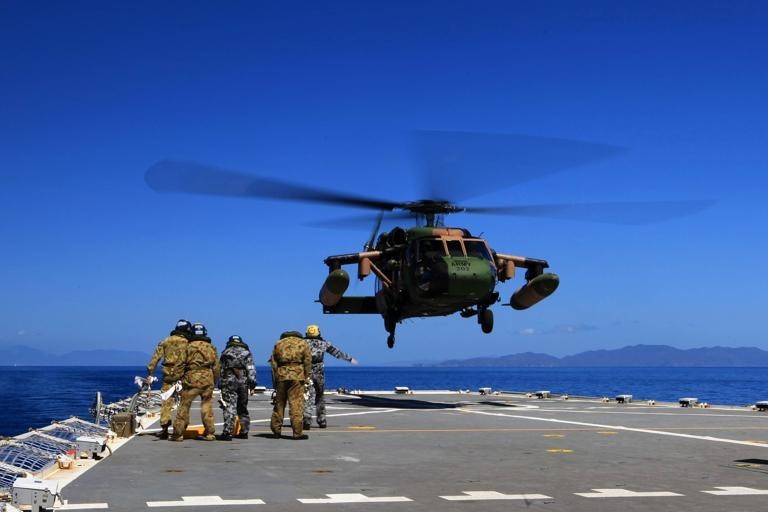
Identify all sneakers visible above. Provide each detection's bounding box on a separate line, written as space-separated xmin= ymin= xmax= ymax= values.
xmin=159 ymin=424 xmax=327 ymax=441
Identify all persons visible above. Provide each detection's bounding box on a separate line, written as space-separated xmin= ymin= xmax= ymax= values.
xmin=218 ymin=335 xmax=257 ymax=441
xmin=143 ymin=318 xmax=192 ymax=440
xmin=303 ymin=325 xmax=357 ymax=429
xmin=169 ymin=323 xmax=220 ymax=442
xmin=271 ymin=331 xmax=312 ymax=439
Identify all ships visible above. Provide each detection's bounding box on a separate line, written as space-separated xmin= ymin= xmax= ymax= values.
xmin=2 ymin=375 xmax=767 ymax=510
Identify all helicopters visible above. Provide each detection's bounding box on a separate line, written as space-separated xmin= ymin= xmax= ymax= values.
xmin=143 ymin=131 xmax=720 ymax=350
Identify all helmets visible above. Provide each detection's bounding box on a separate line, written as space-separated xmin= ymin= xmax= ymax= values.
xmin=176 ymin=319 xmax=320 ymax=342
